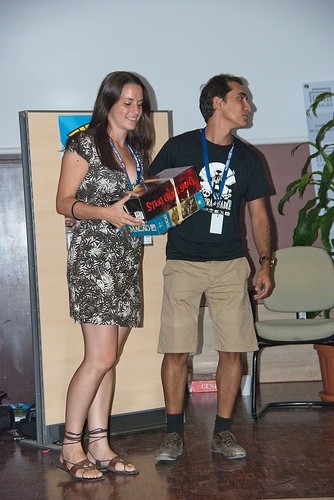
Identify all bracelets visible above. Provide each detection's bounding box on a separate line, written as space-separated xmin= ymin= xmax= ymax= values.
xmin=71 ymin=200 xmax=84 ymax=221
xmin=259 ymin=256 xmax=275 ymax=267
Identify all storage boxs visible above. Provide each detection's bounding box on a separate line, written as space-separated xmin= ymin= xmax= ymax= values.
xmin=188 ymin=373 xmax=218 ymax=393
xmin=109 ymin=165 xmax=201 ymax=229
xmin=126 ymin=191 xmax=205 ymax=236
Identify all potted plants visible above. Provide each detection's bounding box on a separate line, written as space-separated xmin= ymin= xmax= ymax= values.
xmin=278 ymin=92 xmax=334 ymax=408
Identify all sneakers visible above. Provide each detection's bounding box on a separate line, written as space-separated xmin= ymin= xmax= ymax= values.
xmin=153 ymin=433 xmax=183 ymax=460
xmin=210 ymin=431 xmax=246 ymax=460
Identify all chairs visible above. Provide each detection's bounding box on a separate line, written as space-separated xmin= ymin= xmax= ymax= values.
xmin=251 ymin=246 xmax=334 ymax=420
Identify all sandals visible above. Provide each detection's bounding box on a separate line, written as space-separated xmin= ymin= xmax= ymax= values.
xmin=86 ymin=429 xmax=139 ymax=475
xmin=56 ymin=430 xmax=106 ymax=481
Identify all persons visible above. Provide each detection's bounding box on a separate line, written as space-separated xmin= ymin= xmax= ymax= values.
xmin=56 ymin=71 xmax=156 ymax=482
xmin=145 ymin=74 xmax=277 ymax=460
